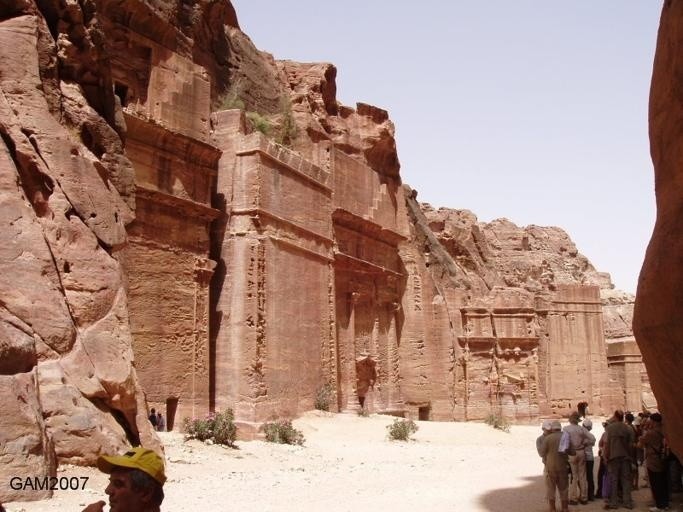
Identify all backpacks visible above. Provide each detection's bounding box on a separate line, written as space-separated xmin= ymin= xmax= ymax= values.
xmin=650 ymin=430 xmax=672 ymax=460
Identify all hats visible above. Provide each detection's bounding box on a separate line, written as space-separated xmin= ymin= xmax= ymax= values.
xmin=568 ymin=412 xmax=583 ymax=422
xmin=542 ymin=421 xmax=552 ymax=430
xmin=552 ymin=420 xmax=561 ymax=429
xmin=651 ymin=413 xmax=662 ymax=422
xmin=583 ymin=419 xmax=592 ymax=430
xmin=97 ymin=448 xmax=166 ymax=487
xmin=602 ymin=419 xmax=614 ymax=427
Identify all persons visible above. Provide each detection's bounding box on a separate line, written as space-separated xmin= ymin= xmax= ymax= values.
xmin=149 ymin=408 xmax=157 ymax=426
xmin=156 ymin=413 xmax=166 ymax=432
xmin=536 ymin=401 xmax=682 ymax=512
xmin=82 ymin=447 xmax=167 ymax=512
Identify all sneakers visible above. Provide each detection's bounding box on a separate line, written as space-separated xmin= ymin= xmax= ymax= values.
xmin=604 ymin=502 xmax=633 ymax=510
xmin=632 ymin=484 xmax=651 ymax=490
xmin=650 ymin=503 xmax=672 ymax=512
xmin=569 ymin=495 xmax=602 ymax=504
